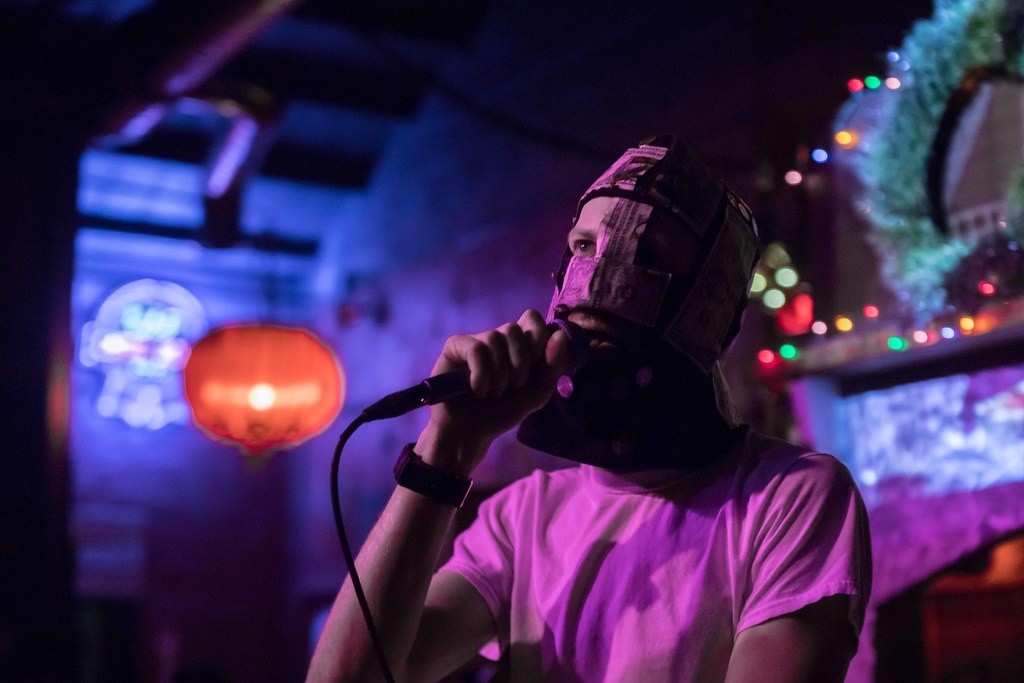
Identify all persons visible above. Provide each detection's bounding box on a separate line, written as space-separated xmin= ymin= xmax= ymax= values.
xmin=305 ymin=133 xmax=873 ymax=683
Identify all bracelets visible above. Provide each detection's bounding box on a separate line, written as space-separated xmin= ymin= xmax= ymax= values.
xmin=393 ymin=443 xmax=473 ymax=507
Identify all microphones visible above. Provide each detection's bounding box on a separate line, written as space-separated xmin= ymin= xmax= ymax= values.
xmin=360 ymin=320 xmax=585 ymax=423
